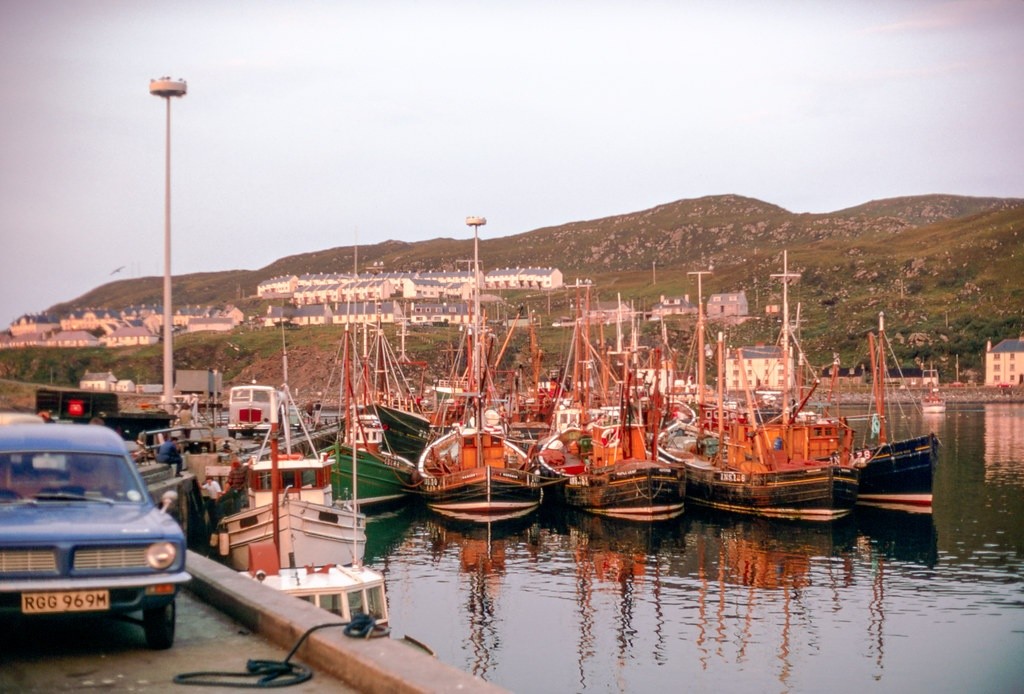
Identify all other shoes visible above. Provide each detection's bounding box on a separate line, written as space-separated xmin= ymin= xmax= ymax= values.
xmin=175 ymin=474 xmax=183 ymax=477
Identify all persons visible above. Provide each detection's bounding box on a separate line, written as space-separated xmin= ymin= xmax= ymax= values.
xmin=180 ymin=403 xmax=195 ymax=438
xmin=306 ymin=400 xmax=321 ymax=422
xmin=156 ymin=436 xmax=184 ymax=477
xmin=198 ymin=459 xmax=252 ymax=499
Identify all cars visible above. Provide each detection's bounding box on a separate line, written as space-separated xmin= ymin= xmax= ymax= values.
xmin=1 ymin=420 xmax=191 ymax=652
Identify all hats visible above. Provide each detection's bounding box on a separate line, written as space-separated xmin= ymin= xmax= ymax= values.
xmin=232 ymin=462 xmax=241 ymax=468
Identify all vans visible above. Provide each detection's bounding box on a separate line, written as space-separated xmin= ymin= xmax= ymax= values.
xmin=226 ymin=384 xmax=288 ymax=439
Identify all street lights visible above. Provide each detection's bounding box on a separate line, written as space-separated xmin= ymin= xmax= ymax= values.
xmin=464 ymin=215 xmax=489 ymax=380
xmin=149 ymin=74 xmax=189 ymax=422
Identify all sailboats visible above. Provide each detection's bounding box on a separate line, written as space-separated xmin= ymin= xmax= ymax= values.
xmin=205 ymin=250 xmax=940 ymax=634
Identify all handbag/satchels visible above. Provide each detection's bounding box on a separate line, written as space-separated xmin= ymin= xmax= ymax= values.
xmin=222 ymin=488 xmax=241 ymax=516
